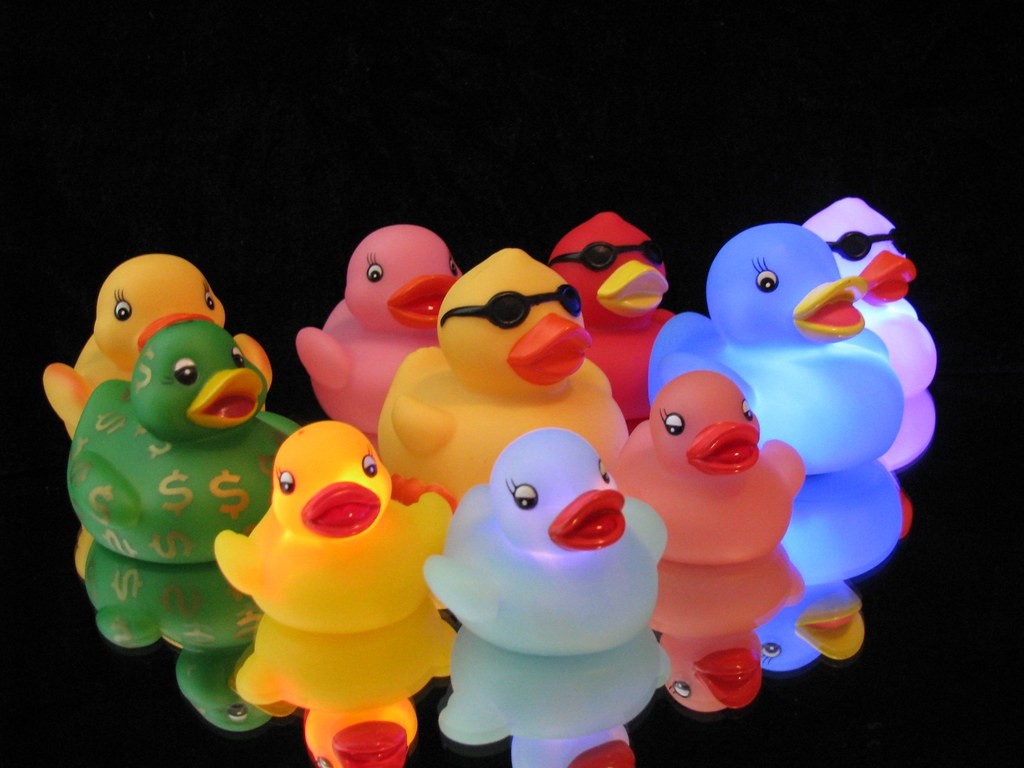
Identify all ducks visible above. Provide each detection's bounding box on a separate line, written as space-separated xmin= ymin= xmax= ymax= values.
xmin=41 ymin=193 xmax=939 ymax=660
xmin=176 ymin=581 xmax=867 ymax=768
